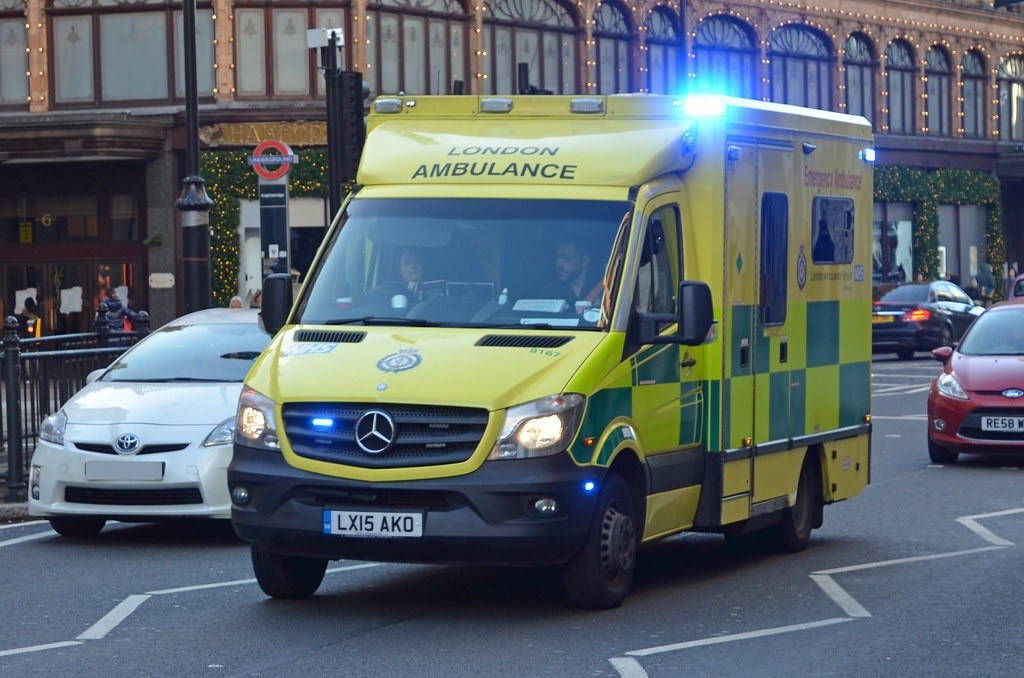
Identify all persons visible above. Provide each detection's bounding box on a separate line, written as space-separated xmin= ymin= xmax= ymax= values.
xmin=230 ymin=296 xmax=242 ymax=308
xmin=18 ymin=297 xmax=39 ymax=383
xmin=398 ymin=249 xmax=443 ymax=301
xmin=1005 ymin=268 xmax=1020 ymax=300
xmin=249 ymin=289 xmax=262 ymax=308
xmin=962 ymin=265 xmax=999 ymax=308
xmin=96 ymin=287 xmax=137 ymax=347
xmin=555 ymin=239 xmax=603 ymax=328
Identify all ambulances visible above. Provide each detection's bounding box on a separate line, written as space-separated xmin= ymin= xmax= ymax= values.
xmin=227 ymin=93 xmax=875 ymax=613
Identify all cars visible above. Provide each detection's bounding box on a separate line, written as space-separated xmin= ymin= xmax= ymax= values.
xmin=925 ymin=298 xmax=1023 ymax=465
xmin=27 ymin=309 xmax=273 ymax=539
xmin=872 ymin=280 xmax=985 ymax=362
